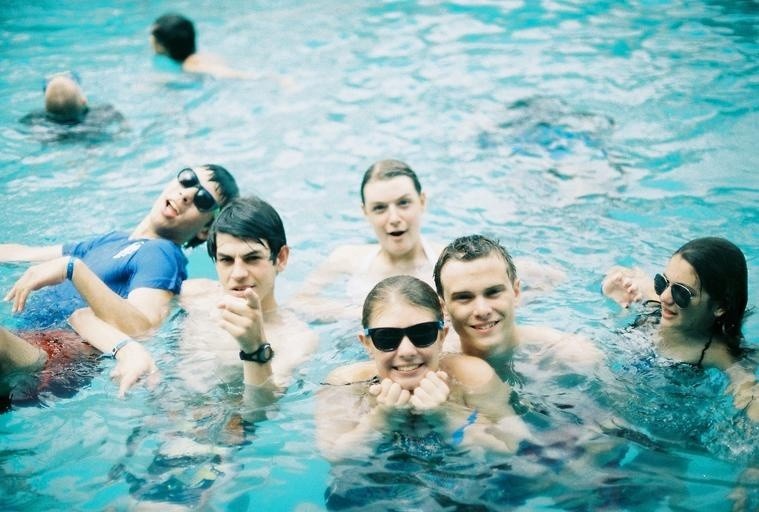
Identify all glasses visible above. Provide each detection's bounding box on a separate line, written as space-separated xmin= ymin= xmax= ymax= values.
xmin=654 ymin=274 xmax=693 ymax=309
xmin=179 ymin=169 xmax=219 ymax=212
xmin=365 ymin=321 xmax=444 ymax=353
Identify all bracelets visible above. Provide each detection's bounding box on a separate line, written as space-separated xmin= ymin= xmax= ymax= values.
xmin=65 ymin=255 xmax=81 ymax=282
xmin=445 ymin=407 xmax=479 ymax=449
xmin=94 ymin=337 xmax=136 ymax=360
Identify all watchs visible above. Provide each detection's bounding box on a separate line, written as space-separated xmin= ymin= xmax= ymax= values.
xmin=239 ymin=341 xmax=275 ymax=365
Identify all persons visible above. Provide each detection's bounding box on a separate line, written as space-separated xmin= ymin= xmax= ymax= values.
xmin=316 ymin=272 xmax=531 ymax=510
xmin=69 ymin=197 xmax=318 ymax=512
xmin=591 ymin=236 xmax=759 ymax=511
xmin=430 ymin=234 xmax=632 ymax=511
xmin=290 ymin=161 xmax=570 ymax=331
xmin=0 ymin=163 xmax=237 ymax=416
xmin=152 ymin=12 xmax=257 ymax=80
xmin=22 ymin=70 xmax=136 ymax=147
